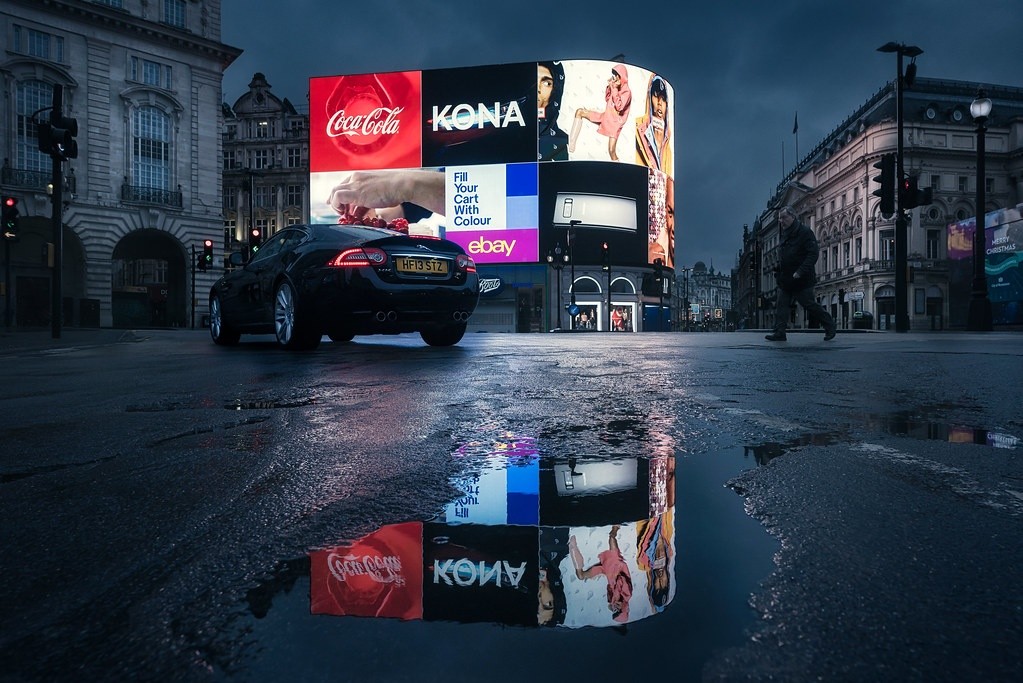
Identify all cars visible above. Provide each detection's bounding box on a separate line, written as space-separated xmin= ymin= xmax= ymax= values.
xmin=207 ymin=223 xmax=481 ymax=351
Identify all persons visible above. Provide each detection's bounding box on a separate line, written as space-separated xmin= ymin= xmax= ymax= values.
xmin=326 ymin=166 xmax=446 ymax=229
xmin=765 ymin=207 xmax=838 ymax=341
xmin=575 ymin=308 xmax=596 ymax=330
xmin=538 ymin=507 xmax=674 ymax=627
xmin=537 ymin=60 xmax=672 ymax=175
xmin=648 ymin=175 xmax=674 ymax=268
xmin=611 ymin=307 xmax=628 ymax=331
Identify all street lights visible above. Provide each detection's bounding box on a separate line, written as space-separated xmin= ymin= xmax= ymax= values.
xmin=545 ymin=242 xmax=570 ymax=333
xmin=682 ymin=267 xmax=695 ymax=331
xmin=876 ymin=41 xmax=925 ymax=336
xmin=601 ymin=242 xmax=611 ymax=332
xmin=960 ymin=84 xmax=996 ymax=333
xmin=568 ymin=219 xmax=582 ymax=330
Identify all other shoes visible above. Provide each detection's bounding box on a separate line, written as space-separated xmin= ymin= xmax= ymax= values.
xmin=824 ymin=323 xmax=838 ymax=340
xmin=765 ymin=332 xmax=788 ymax=341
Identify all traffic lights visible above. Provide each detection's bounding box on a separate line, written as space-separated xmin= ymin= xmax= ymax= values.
xmin=197 ymin=254 xmax=206 ymax=271
xmin=687 ymin=300 xmax=692 ymax=310
xmin=2 ymin=196 xmax=20 ymax=233
xmin=872 ymin=152 xmax=895 ymax=215
xmin=39 ymin=122 xmax=70 ymax=156
xmin=204 ymin=240 xmax=214 ymax=271
xmin=899 ymin=177 xmax=921 ymax=208
xmin=250 ymin=229 xmax=260 ymax=257
xmin=42 ymin=243 xmax=54 ymax=269
xmin=55 ymin=116 xmax=79 ymax=161
xmin=242 ymin=244 xmax=249 ymax=263
xmin=654 ymin=258 xmax=662 ymax=282
